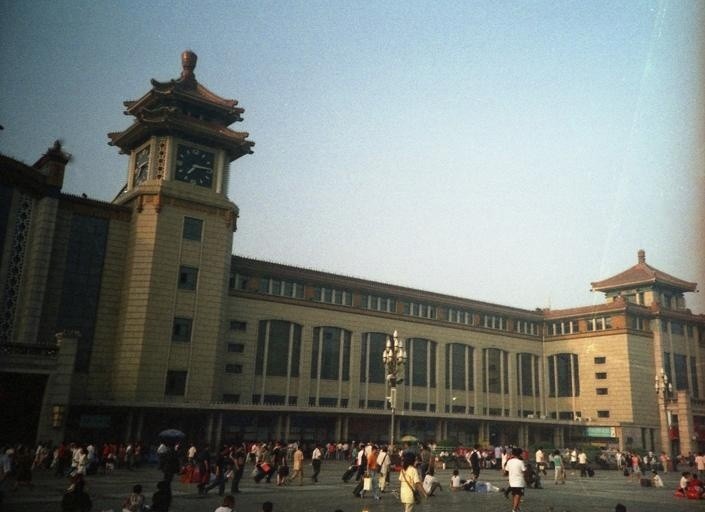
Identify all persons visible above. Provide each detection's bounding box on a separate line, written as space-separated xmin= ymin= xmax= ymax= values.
xmin=311 ymin=440 xmax=592 ymax=511
xmin=597 ymin=446 xmax=704 ymax=511
xmin=2 ymin=436 xmax=304 ymax=511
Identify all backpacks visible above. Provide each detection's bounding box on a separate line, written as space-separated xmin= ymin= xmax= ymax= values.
xmin=470 ymin=450 xmax=480 ymax=465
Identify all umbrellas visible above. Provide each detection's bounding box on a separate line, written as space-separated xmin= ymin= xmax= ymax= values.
xmin=158 ymin=429 xmax=185 ymax=441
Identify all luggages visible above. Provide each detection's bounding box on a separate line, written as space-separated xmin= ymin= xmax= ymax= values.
xmin=342 ymin=469 xmax=353 ymax=482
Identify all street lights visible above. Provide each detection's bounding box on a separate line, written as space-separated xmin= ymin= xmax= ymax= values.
xmin=652 ymin=366 xmax=678 ymax=470
xmin=380 ymin=329 xmax=408 ymax=465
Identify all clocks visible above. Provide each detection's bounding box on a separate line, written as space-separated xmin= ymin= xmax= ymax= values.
xmin=133 ymin=145 xmax=149 ymax=187
xmin=175 ymin=146 xmax=215 ymax=187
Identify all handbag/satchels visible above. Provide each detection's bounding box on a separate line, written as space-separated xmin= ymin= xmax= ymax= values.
xmin=278 ymin=465 xmax=289 ymax=476
xmin=413 ymin=489 xmax=422 ymax=505
xmin=362 ymin=477 xmax=373 ymax=491
xmin=376 ymin=464 xmax=381 ymax=474
